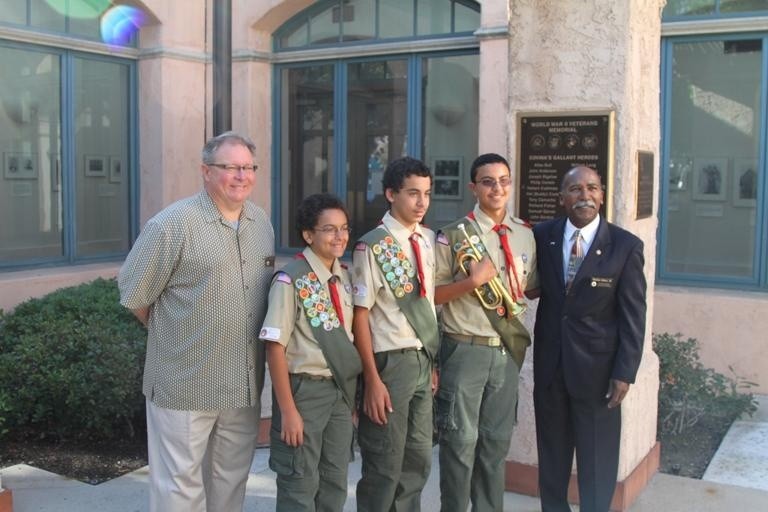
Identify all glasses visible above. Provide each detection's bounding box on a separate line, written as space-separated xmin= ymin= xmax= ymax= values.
xmin=476 ymin=179 xmax=512 ymax=187
xmin=206 ymin=163 xmax=258 ymax=172
xmin=315 ymin=227 xmax=352 ymax=235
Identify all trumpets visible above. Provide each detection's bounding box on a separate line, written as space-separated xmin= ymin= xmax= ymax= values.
xmin=457 ymin=224 xmax=527 ymax=321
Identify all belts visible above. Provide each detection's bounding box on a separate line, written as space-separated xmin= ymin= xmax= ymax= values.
xmin=444 ymin=332 xmax=504 ymax=347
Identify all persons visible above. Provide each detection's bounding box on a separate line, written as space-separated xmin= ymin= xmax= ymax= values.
xmin=116 ymin=131 xmax=276 ymax=512
xmin=351 ymin=155 xmax=440 ymax=511
xmin=256 ymin=192 xmax=360 ymax=511
xmin=433 ymin=153 xmax=542 ymax=512
xmin=526 ymin=163 xmax=647 ymax=511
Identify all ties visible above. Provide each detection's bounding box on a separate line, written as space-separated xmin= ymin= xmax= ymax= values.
xmin=565 ymin=230 xmax=585 ymax=296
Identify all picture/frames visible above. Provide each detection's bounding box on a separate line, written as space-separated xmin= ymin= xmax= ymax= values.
xmin=694 ymin=156 xmax=759 ymax=208
xmin=3 ymin=151 xmax=61 ymax=193
xmin=429 ymin=156 xmax=463 ymax=200
xmin=84 ymin=154 xmax=123 ymax=183
